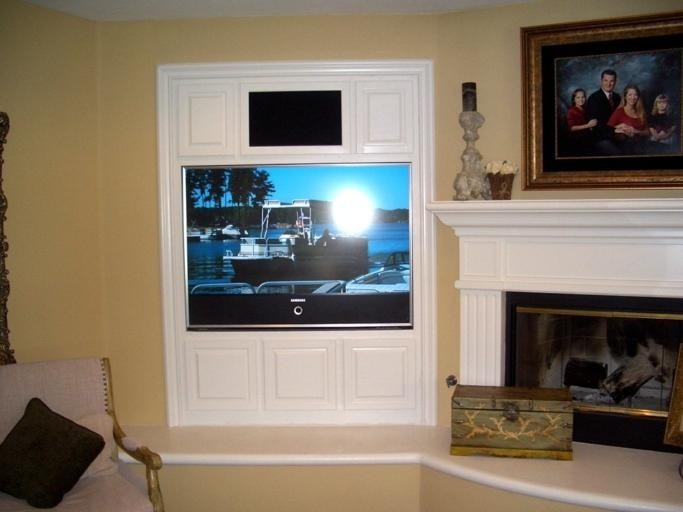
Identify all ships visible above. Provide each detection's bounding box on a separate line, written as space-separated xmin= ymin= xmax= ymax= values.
xmin=221 ymin=201 xmax=368 ymax=286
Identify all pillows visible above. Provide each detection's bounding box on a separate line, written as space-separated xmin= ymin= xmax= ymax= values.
xmin=0 ymin=397 xmax=114 ymax=509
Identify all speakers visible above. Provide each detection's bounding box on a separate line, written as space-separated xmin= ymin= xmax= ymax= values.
xmin=247 ymin=90 xmax=344 ymax=148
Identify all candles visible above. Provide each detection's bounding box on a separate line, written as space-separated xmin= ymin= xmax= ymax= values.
xmin=462 ymin=82 xmax=477 ymax=111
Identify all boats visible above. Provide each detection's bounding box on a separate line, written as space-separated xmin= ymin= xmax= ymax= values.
xmin=189 ymin=221 xmax=248 ymax=239
xmin=345 ymin=251 xmax=409 ymax=294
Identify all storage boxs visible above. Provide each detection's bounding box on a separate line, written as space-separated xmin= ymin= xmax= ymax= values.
xmin=450 ymin=381 xmax=573 ymax=460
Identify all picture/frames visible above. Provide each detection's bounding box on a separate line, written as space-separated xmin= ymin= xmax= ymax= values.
xmin=520 ymin=9 xmax=683 ymax=190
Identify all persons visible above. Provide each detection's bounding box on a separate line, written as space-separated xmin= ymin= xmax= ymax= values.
xmin=565 ymin=69 xmax=676 ymax=157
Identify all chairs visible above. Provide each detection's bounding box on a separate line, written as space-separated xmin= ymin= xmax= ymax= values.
xmin=0 ymin=358 xmax=164 ymax=512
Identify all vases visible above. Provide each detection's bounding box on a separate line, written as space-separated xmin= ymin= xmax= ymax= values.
xmin=487 ymin=174 xmax=514 ymax=200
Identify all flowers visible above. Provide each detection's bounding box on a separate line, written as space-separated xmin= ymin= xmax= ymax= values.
xmin=482 ymin=159 xmax=518 ymax=181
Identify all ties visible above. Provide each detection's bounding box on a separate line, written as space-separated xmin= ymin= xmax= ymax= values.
xmin=608 ymin=93 xmax=613 ymax=108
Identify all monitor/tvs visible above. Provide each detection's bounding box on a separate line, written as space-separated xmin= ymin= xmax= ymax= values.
xmin=182 ymin=161 xmax=414 ymax=332
